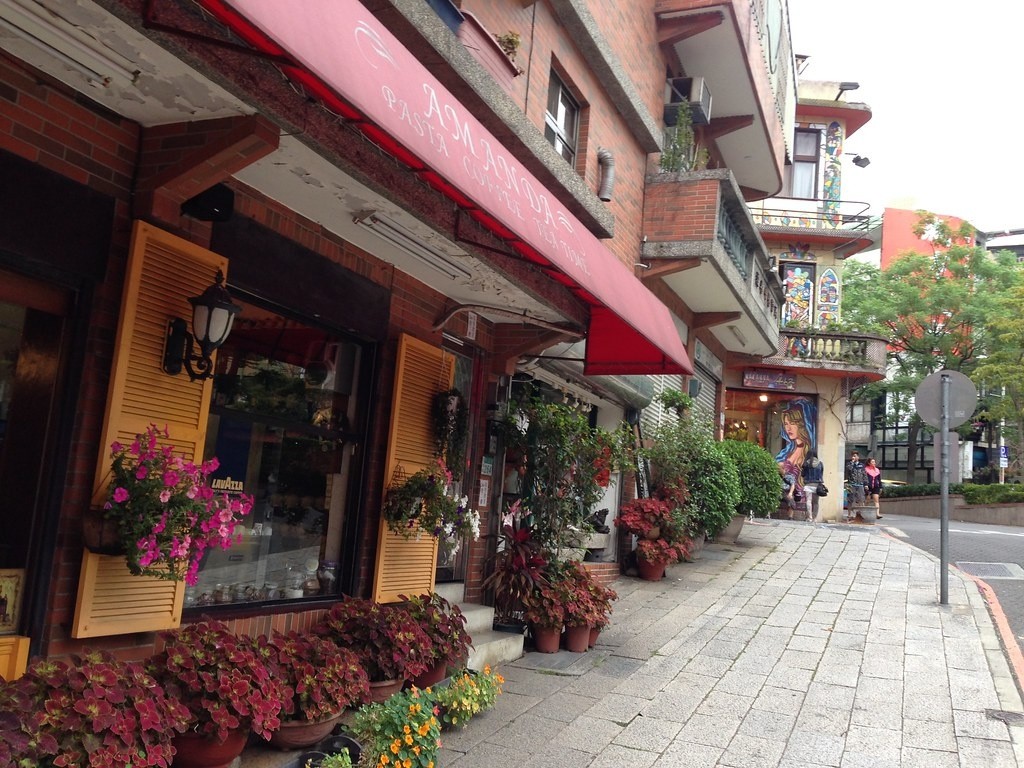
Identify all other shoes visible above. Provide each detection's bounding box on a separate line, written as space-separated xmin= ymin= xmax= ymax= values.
xmin=846 ymin=516 xmax=853 ymax=519
xmin=876 ymin=515 xmax=883 ymax=519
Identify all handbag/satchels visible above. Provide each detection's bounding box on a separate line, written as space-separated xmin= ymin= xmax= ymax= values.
xmin=793 ymin=488 xmax=801 ymax=502
xmin=816 ymin=483 xmax=828 ymax=496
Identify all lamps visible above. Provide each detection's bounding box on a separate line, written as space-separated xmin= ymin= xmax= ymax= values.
xmin=162 ymin=267 xmax=241 ymax=384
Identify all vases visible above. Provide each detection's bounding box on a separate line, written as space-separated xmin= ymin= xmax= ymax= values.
xmin=405 ymin=495 xmax=424 ymax=519
xmin=80 ymin=504 xmax=131 ymax=557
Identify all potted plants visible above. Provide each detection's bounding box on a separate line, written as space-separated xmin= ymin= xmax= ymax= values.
xmin=713 ymin=440 xmax=784 ymax=543
xmin=525 ymin=559 xmax=619 ymax=653
xmin=679 ymin=436 xmax=741 ymax=559
xmin=148 ymin=619 xmax=278 ymax=768
xmin=456 ymin=10 xmax=525 ymax=96
xmin=315 ymin=592 xmax=433 ymax=706
xmin=396 ymin=590 xmax=475 ymax=685
xmin=479 ymin=497 xmax=546 ymax=633
xmin=243 ymin=627 xmax=371 ymax=749
xmin=635 ymin=540 xmax=677 ymax=581
xmin=431 ymin=387 xmax=469 ymax=457
xmin=613 ymin=497 xmax=670 ymax=540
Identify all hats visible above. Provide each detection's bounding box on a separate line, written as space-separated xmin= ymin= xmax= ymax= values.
xmin=851 ymin=450 xmax=859 ymax=455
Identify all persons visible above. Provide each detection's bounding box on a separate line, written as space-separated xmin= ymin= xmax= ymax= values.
xmin=846 ymin=450 xmax=870 ymax=520
xmin=779 ymin=462 xmax=797 ymax=520
xmin=865 ymin=458 xmax=883 ymax=519
xmin=802 ymin=448 xmax=824 ymax=522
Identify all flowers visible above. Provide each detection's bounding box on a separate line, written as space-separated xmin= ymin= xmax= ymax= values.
xmin=110 ymin=421 xmax=253 ymax=585
xmin=305 ymin=662 xmax=504 ymax=768
xmin=384 ymin=459 xmax=481 ymax=559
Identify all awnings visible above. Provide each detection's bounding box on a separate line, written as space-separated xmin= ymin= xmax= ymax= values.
xmin=198 ymin=0 xmax=695 ymax=376
